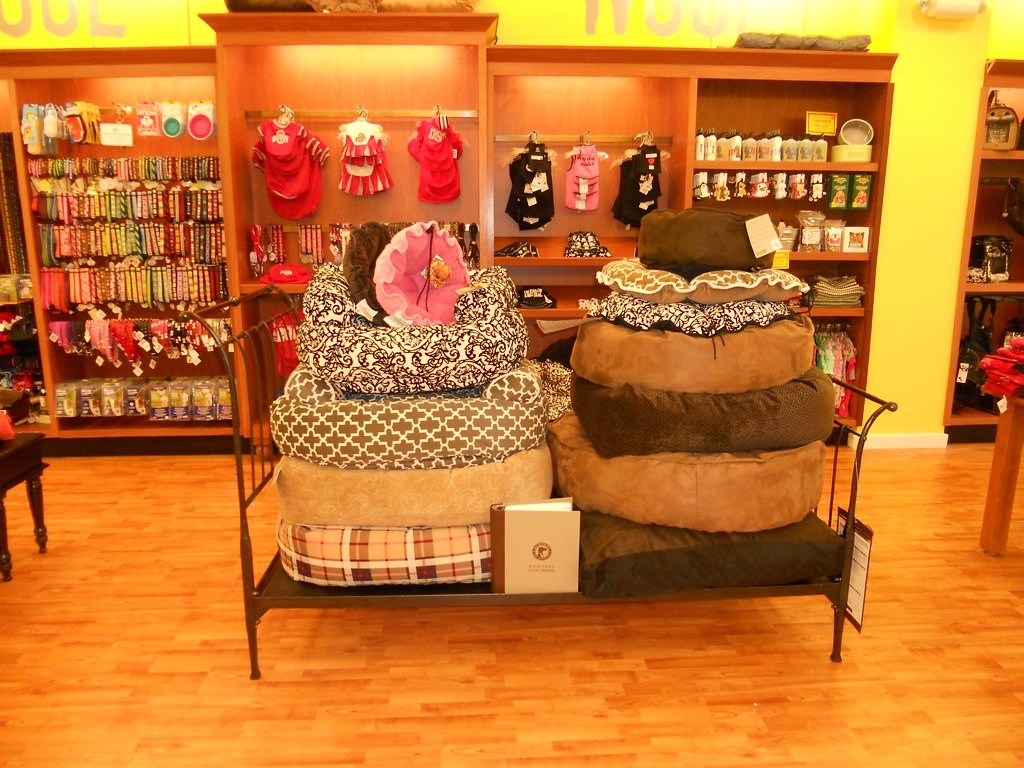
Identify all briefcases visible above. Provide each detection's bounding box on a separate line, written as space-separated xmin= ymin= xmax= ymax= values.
xmin=967 ymin=234 xmax=1012 ymax=283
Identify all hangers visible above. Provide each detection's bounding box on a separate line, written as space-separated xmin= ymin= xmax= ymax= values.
xmin=414 ymin=104 xmax=441 ymax=126
xmin=498 ymin=131 xmax=673 ymax=170
xmin=276 ymin=106 xmax=296 ymax=125
xmin=339 ymin=105 xmax=384 ymax=133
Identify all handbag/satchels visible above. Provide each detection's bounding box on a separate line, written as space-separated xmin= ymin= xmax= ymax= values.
xmin=1002 ymin=178 xmax=1024 ymax=235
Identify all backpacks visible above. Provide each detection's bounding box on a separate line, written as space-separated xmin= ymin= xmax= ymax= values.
xmin=954 ymin=295 xmax=1000 ymax=415
xmin=982 ymin=90 xmax=1020 ymax=151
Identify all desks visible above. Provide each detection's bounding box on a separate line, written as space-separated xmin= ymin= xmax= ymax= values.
xmin=0 ymin=433 xmax=50 ymax=583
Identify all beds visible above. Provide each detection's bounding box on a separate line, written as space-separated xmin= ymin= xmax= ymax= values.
xmin=178 ymin=284 xmax=897 ymax=680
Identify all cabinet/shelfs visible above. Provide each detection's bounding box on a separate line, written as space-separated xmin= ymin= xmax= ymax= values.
xmin=486 ymin=45 xmax=899 ymax=448
xmin=198 ymin=12 xmax=499 ymax=452
xmin=943 ymin=57 xmax=1024 ymax=443
xmin=0 ymin=46 xmax=246 ymax=444
